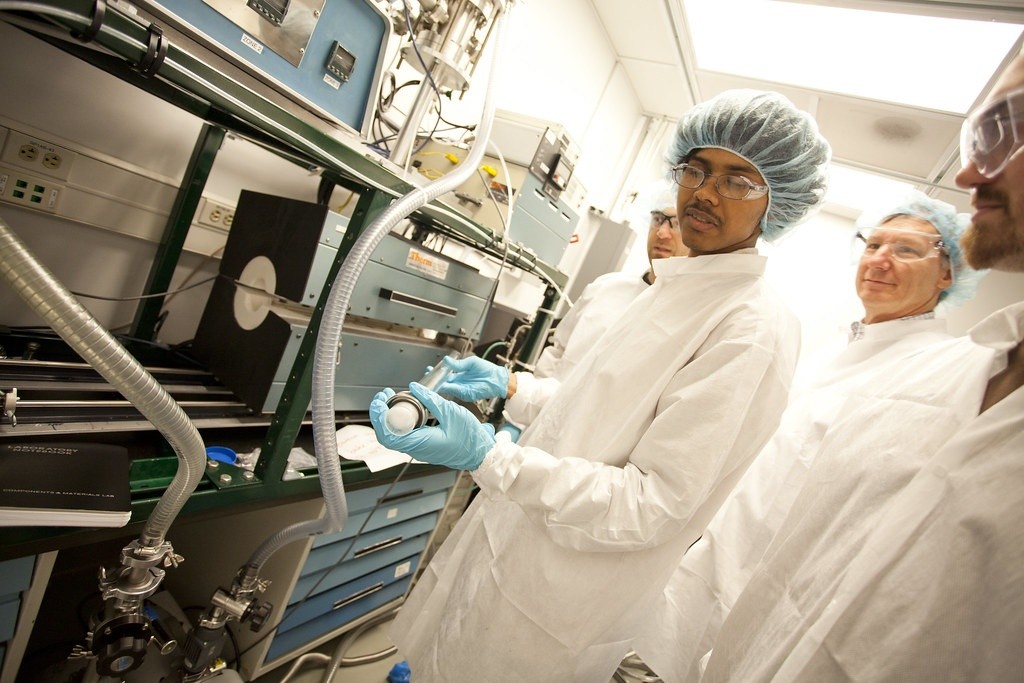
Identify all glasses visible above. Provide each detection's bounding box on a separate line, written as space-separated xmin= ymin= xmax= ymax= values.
xmin=670 ymin=163 xmax=768 ymax=200
xmin=648 ymin=211 xmax=681 ymax=233
xmin=959 ymin=89 xmax=1024 ymax=178
xmin=854 ymin=225 xmax=951 ymax=263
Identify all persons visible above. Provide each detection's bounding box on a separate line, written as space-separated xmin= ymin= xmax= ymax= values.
xmin=698 ymin=46 xmax=1024 ymax=683
xmin=495 ymin=198 xmax=691 ymax=443
xmin=368 ymin=90 xmax=827 ymax=682
xmin=615 ymin=198 xmax=968 ymax=682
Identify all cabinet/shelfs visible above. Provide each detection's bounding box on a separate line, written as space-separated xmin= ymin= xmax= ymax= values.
xmin=0 ymin=1 xmax=573 ymax=683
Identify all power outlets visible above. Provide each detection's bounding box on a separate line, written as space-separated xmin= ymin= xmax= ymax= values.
xmin=197 ymin=199 xmax=235 ymax=231
xmin=2 ymin=130 xmax=78 ymax=181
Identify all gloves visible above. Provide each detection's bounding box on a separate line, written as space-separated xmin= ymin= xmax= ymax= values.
xmin=423 ymin=354 xmax=509 ymax=401
xmin=369 ymin=381 xmax=495 ymax=472
